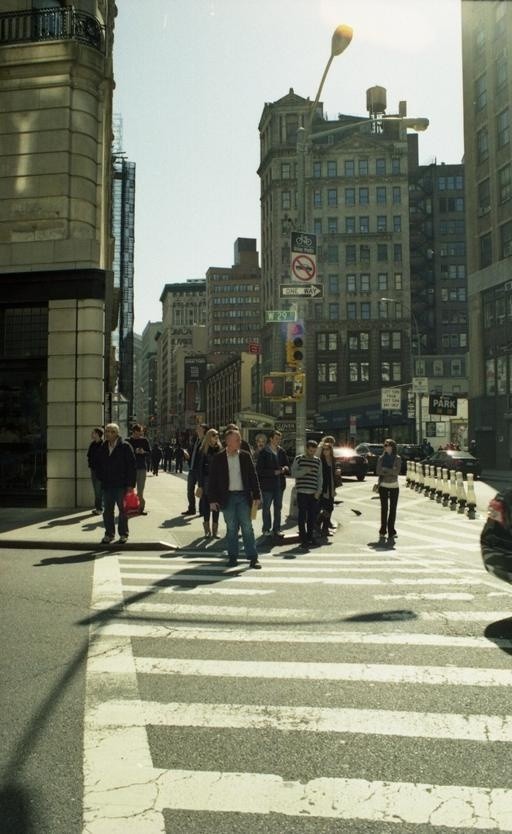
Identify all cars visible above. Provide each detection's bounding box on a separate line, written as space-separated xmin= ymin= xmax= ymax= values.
xmin=418 ymin=449 xmax=482 ymax=479
xmin=480 ymin=488 xmax=512 ymax=585
xmin=332 ymin=444 xmax=369 ymax=482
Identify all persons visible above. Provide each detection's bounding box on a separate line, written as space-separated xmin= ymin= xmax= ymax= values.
xmin=376 ymin=438 xmax=402 ymax=536
xmin=86 ymin=423 xmax=337 ymax=569
xmin=421 ymin=441 xmax=461 ymax=460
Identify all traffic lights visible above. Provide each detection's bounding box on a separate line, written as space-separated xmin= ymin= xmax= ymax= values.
xmin=286 ymin=321 xmax=304 ymax=366
xmin=261 ymin=371 xmax=288 ymax=400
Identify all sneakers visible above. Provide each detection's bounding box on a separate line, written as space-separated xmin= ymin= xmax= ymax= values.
xmin=227 ymin=557 xmax=237 ymax=566
xmin=262 ymin=528 xmax=284 ymax=537
xmin=119 ymin=535 xmax=128 ymax=542
xmin=249 ymin=560 xmax=262 ymax=569
xmin=102 ymin=535 xmax=113 ymax=542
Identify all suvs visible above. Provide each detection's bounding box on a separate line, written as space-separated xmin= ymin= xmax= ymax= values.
xmin=393 ymin=443 xmax=427 ymax=475
xmin=351 ymin=441 xmax=384 ymax=475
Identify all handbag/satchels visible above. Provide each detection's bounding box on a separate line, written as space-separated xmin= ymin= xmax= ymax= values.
xmin=123 ymin=493 xmax=141 ymax=517
xmin=373 ymin=484 xmax=378 ymax=493
xmin=93 ymin=510 xmax=102 ymax=515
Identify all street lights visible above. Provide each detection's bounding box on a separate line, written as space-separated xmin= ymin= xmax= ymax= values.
xmin=379 ymin=295 xmax=425 ymax=444
xmin=287 ymin=16 xmax=431 ymax=456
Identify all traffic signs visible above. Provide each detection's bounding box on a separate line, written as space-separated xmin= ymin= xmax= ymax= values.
xmin=273 ymin=419 xmax=315 ymax=432
xmin=279 ymin=283 xmax=326 ymax=299
xmin=264 ymin=309 xmax=298 ymax=323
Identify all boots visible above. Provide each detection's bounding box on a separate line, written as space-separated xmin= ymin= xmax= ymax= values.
xmin=202 ymin=521 xmax=220 ymax=539
